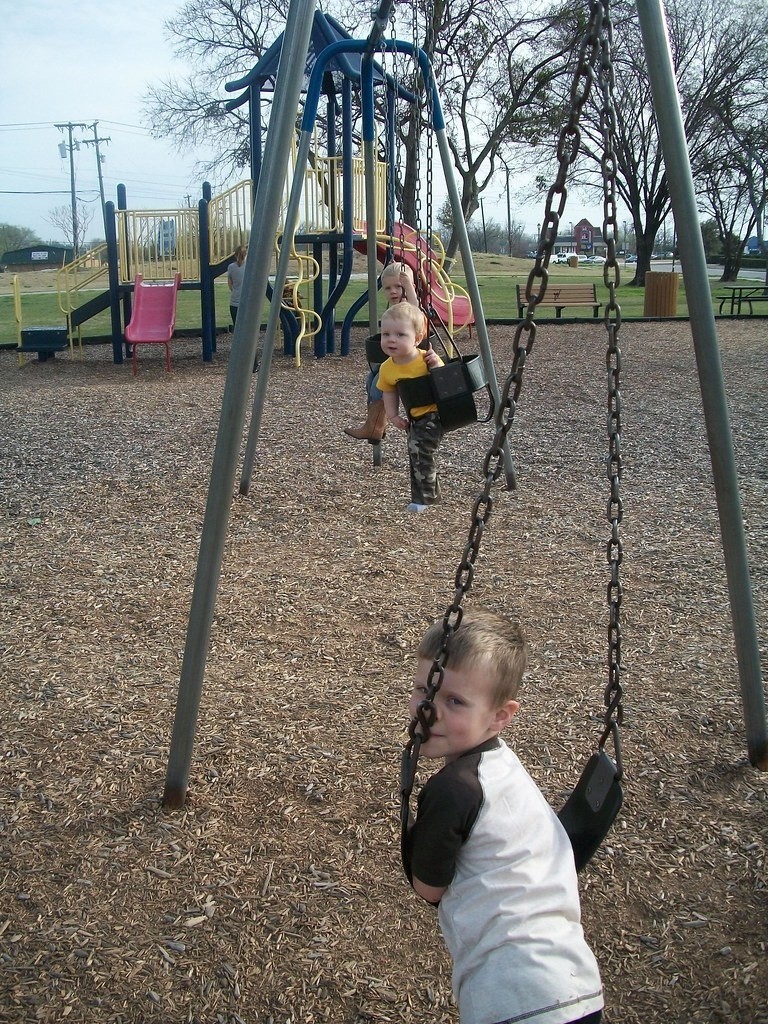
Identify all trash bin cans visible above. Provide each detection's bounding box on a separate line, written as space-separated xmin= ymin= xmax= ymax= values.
xmin=570 ymin=255 xmax=579 ymax=268
xmin=643 ymin=270 xmax=679 ymax=318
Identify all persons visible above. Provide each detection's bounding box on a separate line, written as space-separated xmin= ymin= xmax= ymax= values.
xmin=400 ymin=610 xmax=603 ymax=1024
xmin=282 ymin=279 xmax=302 ymax=332
xmin=227 ymin=245 xmax=260 ymax=373
xmin=344 ymin=261 xmax=445 ymax=513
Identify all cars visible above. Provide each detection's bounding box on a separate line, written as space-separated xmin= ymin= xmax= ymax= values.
xmin=585 ymin=255 xmax=605 ymax=263
xmin=626 ymin=256 xmax=639 ymax=263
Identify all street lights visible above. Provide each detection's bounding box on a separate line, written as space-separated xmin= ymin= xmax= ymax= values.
xmin=569 ymin=221 xmax=573 ymax=252
xmin=623 ymin=220 xmax=627 ymax=259
xmin=537 ymin=223 xmax=541 ymax=250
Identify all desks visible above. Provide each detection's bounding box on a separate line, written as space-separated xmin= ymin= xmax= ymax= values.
xmin=716 ymin=286 xmax=768 ymax=314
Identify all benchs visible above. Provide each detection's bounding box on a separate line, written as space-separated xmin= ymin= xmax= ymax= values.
xmin=516 ymin=283 xmax=603 ymax=318
xmin=716 ymin=296 xmax=768 ymax=301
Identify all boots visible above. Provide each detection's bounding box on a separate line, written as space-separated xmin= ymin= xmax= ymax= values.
xmin=344 ymin=399 xmax=387 ymax=446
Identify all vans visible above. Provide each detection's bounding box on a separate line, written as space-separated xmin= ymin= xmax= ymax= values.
xmin=574 ymin=255 xmax=588 ymax=261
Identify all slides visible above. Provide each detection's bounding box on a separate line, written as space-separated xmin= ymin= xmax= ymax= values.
xmin=353 ymin=224 xmax=476 ymax=328
xmin=124 ymin=274 xmax=178 ymax=344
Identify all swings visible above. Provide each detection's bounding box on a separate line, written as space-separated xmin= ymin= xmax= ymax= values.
xmin=368 ymin=2 xmax=496 ymax=431
xmin=396 ymin=0 xmax=629 ymax=909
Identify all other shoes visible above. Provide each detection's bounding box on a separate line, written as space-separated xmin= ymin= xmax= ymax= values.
xmin=253 ymin=367 xmax=259 ymax=373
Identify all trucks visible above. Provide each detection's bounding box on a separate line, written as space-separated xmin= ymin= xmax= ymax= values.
xmin=541 ymin=253 xmax=577 ymax=263
